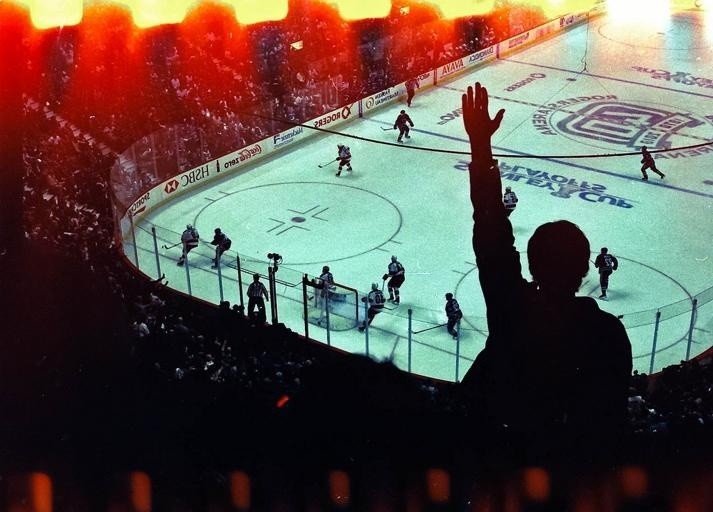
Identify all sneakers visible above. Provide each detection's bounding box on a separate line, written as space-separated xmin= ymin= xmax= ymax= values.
xmin=661 ymin=174 xmax=664 ymax=179
xmin=642 ymin=177 xmax=648 ymax=180
xmin=359 ymin=327 xmax=365 ymax=331
xmin=599 ymin=294 xmax=606 ymax=297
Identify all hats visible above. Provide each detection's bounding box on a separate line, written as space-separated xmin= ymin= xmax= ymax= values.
xmin=253 ymin=273 xmax=261 ymax=278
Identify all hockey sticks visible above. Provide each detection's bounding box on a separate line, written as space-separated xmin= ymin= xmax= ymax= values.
xmin=165 ymin=242 xmax=181 ymax=250
xmin=385 ymin=306 xmax=398 ymax=311
xmin=410 ymin=323 xmax=447 ymax=335
xmin=381 ymin=127 xmax=394 ymax=131
xmin=318 ymin=160 xmax=337 ymax=168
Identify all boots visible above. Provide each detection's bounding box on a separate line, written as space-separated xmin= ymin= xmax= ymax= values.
xmin=347 ymin=167 xmax=352 ymax=170
xmin=336 ymin=173 xmax=340 ymax=176
xmin=398 ymin=137 xmax=403 ymax=142
xmin=405 ymin=133 xmax=410 ymax=138
xmin=178 ymin=261 xmax=184 ymax=265
xmin=389 ymin=297 xmax=399 ymax=303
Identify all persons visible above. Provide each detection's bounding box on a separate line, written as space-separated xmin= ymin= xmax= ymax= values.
xmin=1 ymin=184 xmax=152 ymax=510
xmin=1 ymin=0 xmax=558 ymax=184
xmin=502 ymin=185 xmax=519 ymax=219
xmin=318 ymin=264 xmax=334 ymax=308
xmin=639 ymin=143 xmax=665 ymax=182
xmin=174 ymin=223 xmax=199 ymax=266
xmin=153 ymin=273 xmax=712 ymax=512
xmin=593 ymin=247 xmax=617 ymax=299
xmin=382 ymin=254 xmax=405 ymax=303
xmin=208 ymin=227 xmax=232 ymax=269
xmin=452 ymin=79 xmax=632 ymax=470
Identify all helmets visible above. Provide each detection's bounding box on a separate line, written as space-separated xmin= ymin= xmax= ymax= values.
xmin=215 ymin=228 xmax=220 ymax=233
xmin=187 ymin=224 xmax=192 ymax=229
xmin=446 ymin=293 xmax=452 ymax=298
xmin=506 ymin=187 xmax=511 ymax=191
xmin=601 ymin=248 xmax=607 ymax=252
xmin=392 ymin=255 xmax=397 ymax=261
xmin=372 ymin=282 xmax=378 ymax=289
xmin=323 ymin=266 xmax=329 ymax=272
